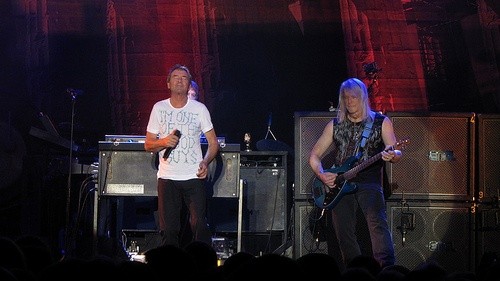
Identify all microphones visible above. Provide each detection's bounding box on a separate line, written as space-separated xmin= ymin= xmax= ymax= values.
xmin=315 ymin=224 xmax=321 ymax=246
xmin=402 ymin=215 xmax=408 ymax=245
xmin=66 ymin=88 xmax=76 ymax=98
xmin=163 ymin=130 xmax=180 ymax=158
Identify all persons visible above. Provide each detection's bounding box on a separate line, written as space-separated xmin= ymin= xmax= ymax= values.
xmin=144 ymin=64 xmax=219 ymax=248
xmin=309 ymin=78 xmax=402 ymax=273
xmin=240 ymin=131 xmax=257 ymax=151
xmin=0 ymin=234 xmax=500 ymax=281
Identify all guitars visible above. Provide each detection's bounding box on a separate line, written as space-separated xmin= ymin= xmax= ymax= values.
xmin=311 ymin=136 xmax=411 ymax=208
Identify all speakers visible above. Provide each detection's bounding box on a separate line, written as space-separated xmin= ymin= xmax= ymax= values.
xmin=212 ymin=149 xmax=288 ymax=234
xmin=293 ymin=112 xmax=500 ymax=270
